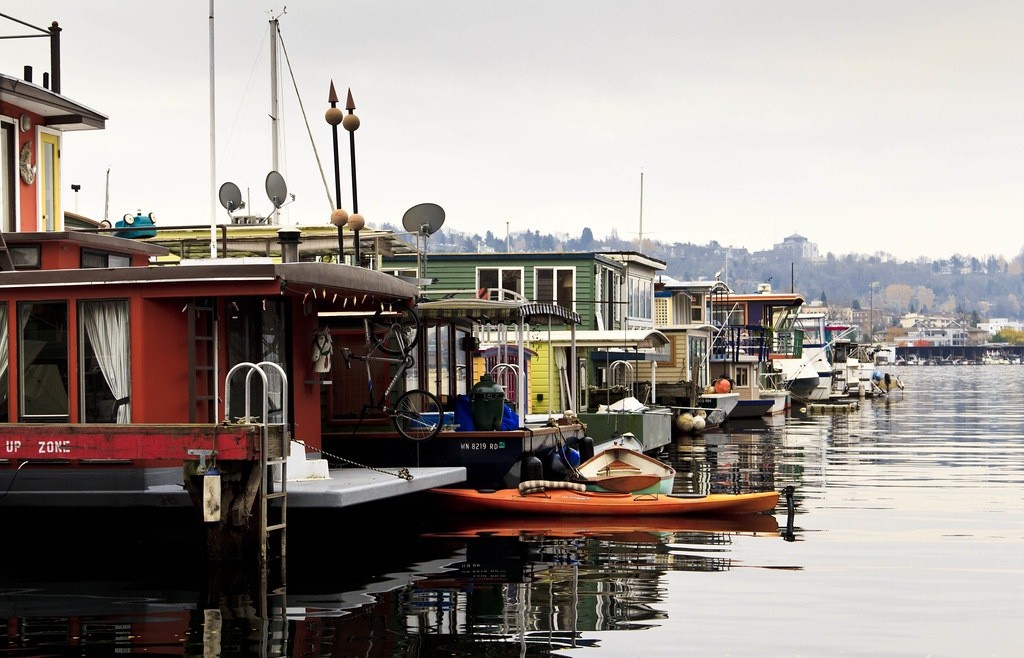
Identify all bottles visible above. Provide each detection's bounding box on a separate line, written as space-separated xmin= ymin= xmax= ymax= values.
xmin=469 ymin=374 xmax=505 ymax=431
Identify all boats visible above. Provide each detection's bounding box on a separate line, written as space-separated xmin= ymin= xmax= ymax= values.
xmin=0 ymin=2 xmax=903 ymax=519
xmin=423 ymin=514 xmax=784 ymax=538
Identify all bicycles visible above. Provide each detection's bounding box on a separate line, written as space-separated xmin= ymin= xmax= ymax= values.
xmin=341 ymin=300 xmax=444 ymax=441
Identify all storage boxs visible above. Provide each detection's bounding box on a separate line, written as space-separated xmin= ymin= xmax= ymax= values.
xmin=412 ymin=412 xmax=454 ymax=427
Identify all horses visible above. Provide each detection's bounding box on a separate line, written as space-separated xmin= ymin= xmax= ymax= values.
xmin=311 ymin=325 xmax=333 ymax=373
